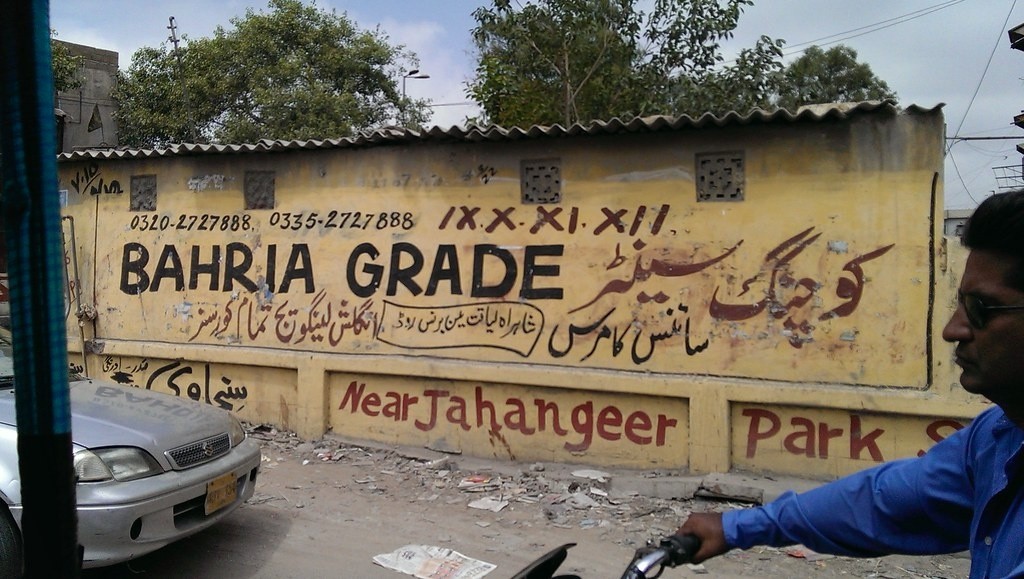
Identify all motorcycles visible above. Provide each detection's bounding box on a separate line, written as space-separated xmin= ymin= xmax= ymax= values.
xmin=511 ymin=532 xmax=701 ymax=579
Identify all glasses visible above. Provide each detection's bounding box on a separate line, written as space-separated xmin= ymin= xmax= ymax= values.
xmin=957 ymin=289 xmax=1024 ymax=330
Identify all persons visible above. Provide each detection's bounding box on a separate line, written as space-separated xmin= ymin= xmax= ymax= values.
xmin=677 ymin=189 xmax=1024 ymax=579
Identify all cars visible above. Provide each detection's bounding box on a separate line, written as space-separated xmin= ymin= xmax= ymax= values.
xmin=0 ymin=323 xmax=262 ymax=579
